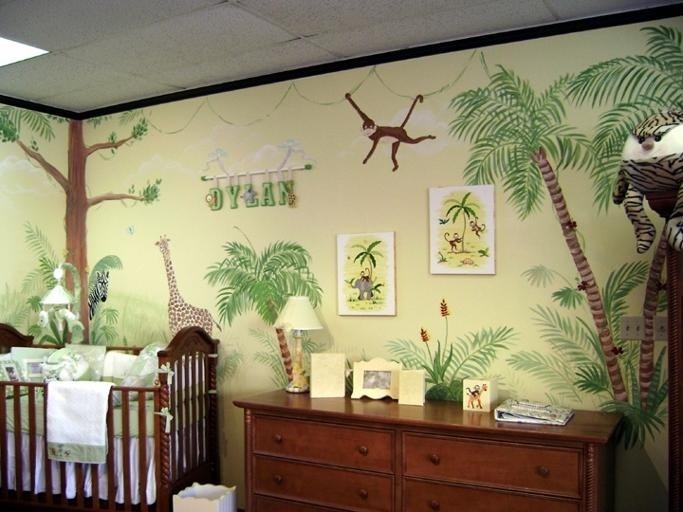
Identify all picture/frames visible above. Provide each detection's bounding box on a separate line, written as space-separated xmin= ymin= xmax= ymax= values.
xmin=338 ymin=231 xmax=396 ymax=315
xmin=429 ymin=184 xmax=495 ymax=275
xmin=0 ymin=360 xmax=29 ymax=398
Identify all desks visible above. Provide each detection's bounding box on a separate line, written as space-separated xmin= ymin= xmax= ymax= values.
xmin=233 ymin=390 xmax=624 ymax=512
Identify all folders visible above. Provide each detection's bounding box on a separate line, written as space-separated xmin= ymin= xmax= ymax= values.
xmin=494 ymin=398 xmax=575 ymax=426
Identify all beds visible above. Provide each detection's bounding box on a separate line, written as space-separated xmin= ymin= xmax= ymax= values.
xmin=0 ymin=324 xmax=220 ymax=512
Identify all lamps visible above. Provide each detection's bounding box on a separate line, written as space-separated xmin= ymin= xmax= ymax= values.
xmin=273 ymin=296 xmax=323 ymax=392
xmin=40 ymin=262 xmax=85 ymax=345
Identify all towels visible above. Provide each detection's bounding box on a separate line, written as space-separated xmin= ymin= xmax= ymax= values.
xmin=46 ymin=382 xmax=114 ymax=464
